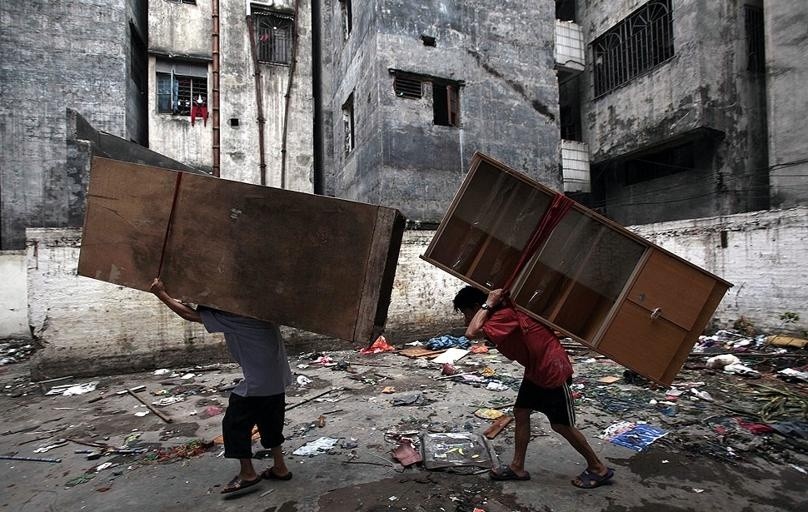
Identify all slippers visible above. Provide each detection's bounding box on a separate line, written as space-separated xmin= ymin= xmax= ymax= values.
xmin=488 ymin=464 xmax=531 ymax=481
xmin=258 ymin=467 xmax=293 ymax=480
xmin=570 ymin=468 xmax=614 ymax=489
xmin=220 ymin=475 xmax=263 ymax=497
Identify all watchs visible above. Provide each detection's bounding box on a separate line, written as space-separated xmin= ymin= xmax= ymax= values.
xmin=480 ymin=303 xmax=494 ymax=314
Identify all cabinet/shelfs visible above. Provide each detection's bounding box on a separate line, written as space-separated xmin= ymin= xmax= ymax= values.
xmin=76 ymin=155 xmax=407 ymax=348
xmin=419 ymin=149 xmax=734 ymax=391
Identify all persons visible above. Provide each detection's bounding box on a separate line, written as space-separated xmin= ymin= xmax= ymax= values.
xmin=148 ymin=276 xmax=293 ymax=498
xmin=453 ymin=285 xmax=614 ymax=490
